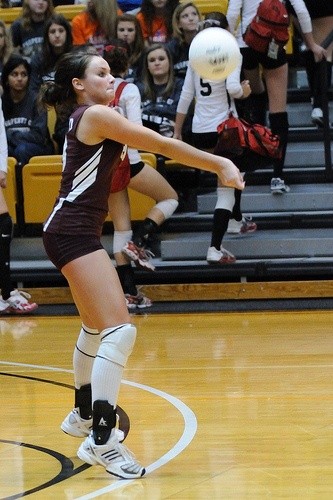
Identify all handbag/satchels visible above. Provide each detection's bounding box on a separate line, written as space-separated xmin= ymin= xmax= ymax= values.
xmin=216 ymin=116 xmax=282 ymax=171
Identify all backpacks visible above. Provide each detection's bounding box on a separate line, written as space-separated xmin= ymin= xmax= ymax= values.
xmin=242 ymin=0 xmax=291 ymax=57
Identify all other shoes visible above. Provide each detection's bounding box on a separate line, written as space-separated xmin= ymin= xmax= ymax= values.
xmin=270 ymin=177 xmax=290 ymax=194
xmin=310 ymin=106 xmax=331 ymax=129
xmin=61 ymin=408 xmax=125 ymax=443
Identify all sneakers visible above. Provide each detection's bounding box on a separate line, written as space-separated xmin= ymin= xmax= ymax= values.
xmin=206 ymin=244 xmax=235 ymax=264
xmin=121 ymin=241 xmax=157 ymax=273
xmin=226 ymin=214 xmax=257 ymax=234
xmin=124 ymin=290 xmax=153 ymax=309
xmin=77 ymin=427 xmax=146 ymax=480
xmin=0 ymin=290 xmax=39 ymax=314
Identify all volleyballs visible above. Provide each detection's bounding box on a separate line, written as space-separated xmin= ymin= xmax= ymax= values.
xmin=189 ymin=27 xmax=240 ymax=81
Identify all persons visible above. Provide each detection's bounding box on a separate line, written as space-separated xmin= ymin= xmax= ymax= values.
xmin=0 ymin=0 xmax=201 ymax=177
xmin=172 ymin=12 xmax=258 ymax=266
xmin=105 ymin=44 xmax=178 ymax=309
xmin=43 ymin=53 xmax=245 ymax=478
xmin=226 ymin=0 xmax=333 ymax=195
xmin=0 ymin=74 xmax=39 ymax=315
